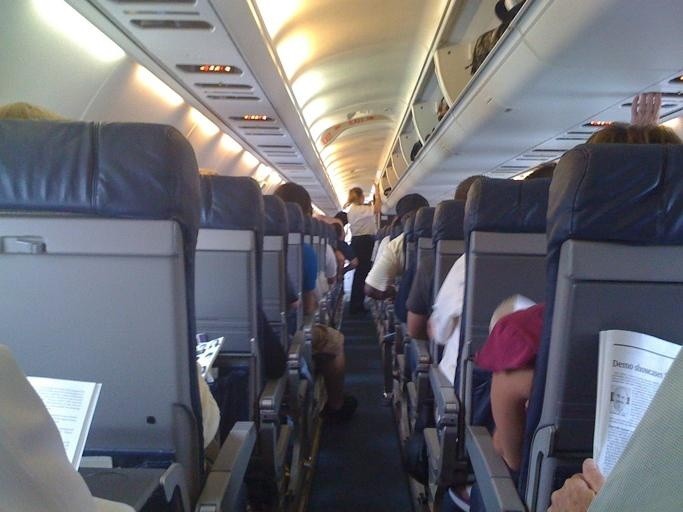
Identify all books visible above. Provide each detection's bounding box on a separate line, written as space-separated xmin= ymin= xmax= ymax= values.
xmin=193 ymin=335 xmax=225 ymax=384
xmin=24 ymin=372 xmax=103 ymax=477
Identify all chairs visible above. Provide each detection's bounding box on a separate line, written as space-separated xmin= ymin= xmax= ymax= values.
xmin=2 ymin=119 xmax=338 ymax=512
xmin=372 ymin=118 xmax=682 ymax=512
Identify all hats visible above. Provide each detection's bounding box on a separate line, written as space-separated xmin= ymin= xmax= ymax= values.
xmin=390 ymin=193 xmax=429 ymax=226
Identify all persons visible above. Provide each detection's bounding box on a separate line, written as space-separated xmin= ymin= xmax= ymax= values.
xmin=191 ymin=327 xmax=226 ymax=476
xmin=343 ymin=182 xmax=382 ymax=313
xmin=256 ymin=179 xmax=358 ymax=420
xmin=361 ymin=193 xmax=430 ymax=300
xmin=404 ymin=161 xmax=558 ymax=390
xmin=477 ymin=93 xmax=680 ymax=512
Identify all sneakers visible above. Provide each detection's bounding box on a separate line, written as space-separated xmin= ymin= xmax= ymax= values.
xmin=447 ymin=484 xmax=472 ymax=511
xmin=324 ymin=395 xmax=358 ymax=424
